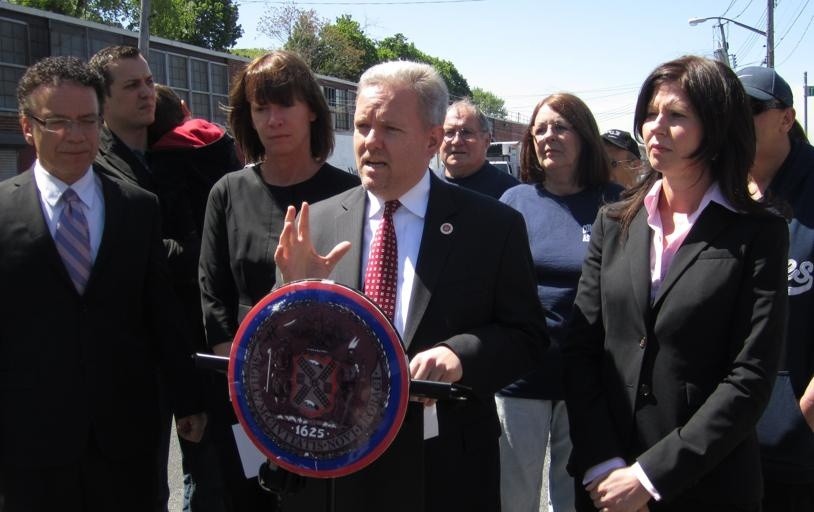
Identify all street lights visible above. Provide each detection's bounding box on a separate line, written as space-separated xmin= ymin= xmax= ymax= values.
xmin=689 ymin=15 xmax=775 ymax=69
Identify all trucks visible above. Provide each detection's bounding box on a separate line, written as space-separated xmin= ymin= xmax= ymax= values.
xmin=488 ymin=141 xmax=523 ymax=183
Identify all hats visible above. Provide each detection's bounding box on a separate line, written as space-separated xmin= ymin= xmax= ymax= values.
xmin=734 ymin=67 xmax=793 ymax=107
xmin=600 ymin=130 xmax=641 ymax=160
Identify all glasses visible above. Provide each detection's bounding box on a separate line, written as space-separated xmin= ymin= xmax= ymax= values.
xmin=531 ymin=123 xmax=572 ymax=137
xmin=30 ymin=114 xmax=105 ymax=135
xmin=443 ymin=126 xmax=475 ymax=141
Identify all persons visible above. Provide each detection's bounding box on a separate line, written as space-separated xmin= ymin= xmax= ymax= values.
xmin=559 ymin=55 xmax=791 ymax=511
xmin=431 ymin=100 xmax=523 ymax=200
xmin=600 ymin=129 xmax=644 ymax=191
xmin=494 ymin=92 xmax=634 ymax=512
xmin=736 ymin=66 xmax=814 ymax=512
xmin=88 ymin=46 xmax=249 ymax=512
xmin=198 ymin=50 xmax=363 ymax=511
xmin=1 ymin=55 xmax=207 ymax=511
xmin=271 ymin=60 xmax=548 ymax=511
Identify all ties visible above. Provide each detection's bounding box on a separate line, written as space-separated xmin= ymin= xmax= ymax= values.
xmin=53 ymin=188 xmax=92 ymax=295
xmin=362 ymin=198 xmax=403 ymax=325
